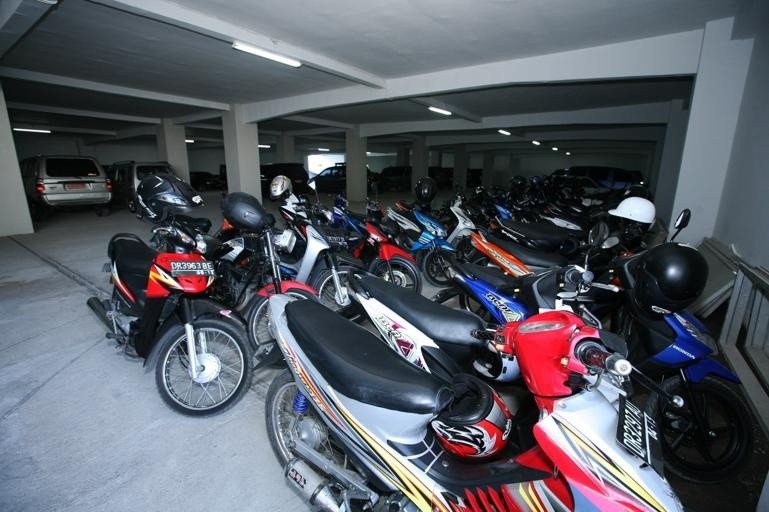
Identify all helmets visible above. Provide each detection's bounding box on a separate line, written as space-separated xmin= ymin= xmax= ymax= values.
xmin=414 ymin=176 xmax=439 ymax=204
xmin=133 ymin=171 xmax=207 ymax=225
xmin=219 ymin=191 xmax=266 ymax=234
xmin=529 ymin=175 xmax=546 ymax=190
xmin=631 ymin=242 xmax=711 ymax=320
xmin=266 ymin=174 xmax=294 ymax=200
xmin=510 ymin=175 xmax=528 ymax=190
xmin=623 ymin=183 xmax=655 ymax=204
xmin=607 ymin=196 xmax=657 ymax=231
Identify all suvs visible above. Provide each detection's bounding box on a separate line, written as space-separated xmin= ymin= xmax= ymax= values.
xmin=18 ymin=152 xmax=115 ymax=222
